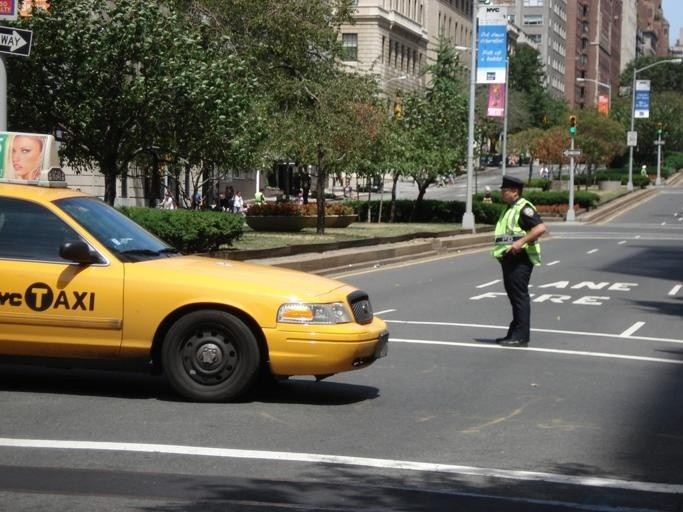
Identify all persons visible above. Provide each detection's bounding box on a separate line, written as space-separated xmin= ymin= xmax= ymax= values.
xmin=641 ymin=165 xmax=648 ymax=178
xmin=150 ymin=185 xmax=244 ymax=213
xmin=299 ymin=187 xmax=304 ymax=206
xmin=303 ymin=183 xmax=309 ymax=208
xmin=4 ymin=134 xmax=47 ymax=181
xmin=255 ymin=189 xmax=265 ymax=208
xmin=492 ymin=176 xmax=545 ymax=347
xmin=540 ymin=165 xmax=548 ymax=179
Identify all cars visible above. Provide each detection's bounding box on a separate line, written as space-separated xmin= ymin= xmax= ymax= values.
xmin=0 ymin=132 xmax=389 ymax=403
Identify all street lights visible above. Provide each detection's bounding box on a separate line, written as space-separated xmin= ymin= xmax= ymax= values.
xmin=576 ymin=77 xmax=611 ymax=113
xmin=626 ymin=58 xmax=682 ymax=191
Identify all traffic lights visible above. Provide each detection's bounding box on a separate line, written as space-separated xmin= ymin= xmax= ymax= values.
xmin=657 ymin=122 xmax=663 ymax=134
xmin=568 ymin=115 xmax=577 ymax=136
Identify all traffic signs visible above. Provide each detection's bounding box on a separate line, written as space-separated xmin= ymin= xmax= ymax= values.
xmin=653 ymin=140 xmax=665 ymax=145
xmin=564 ymin=149 xmax=583 ymax=158
xmin=0 ymin=25 xmax=33 ymax=57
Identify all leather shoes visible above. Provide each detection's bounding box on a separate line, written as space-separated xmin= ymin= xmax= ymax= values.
xmin=496 ymin=338 xmax=528 ymax=347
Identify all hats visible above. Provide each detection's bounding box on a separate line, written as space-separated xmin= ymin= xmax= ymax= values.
xmin=501 ymin=175 xmax=524 ymax=189
